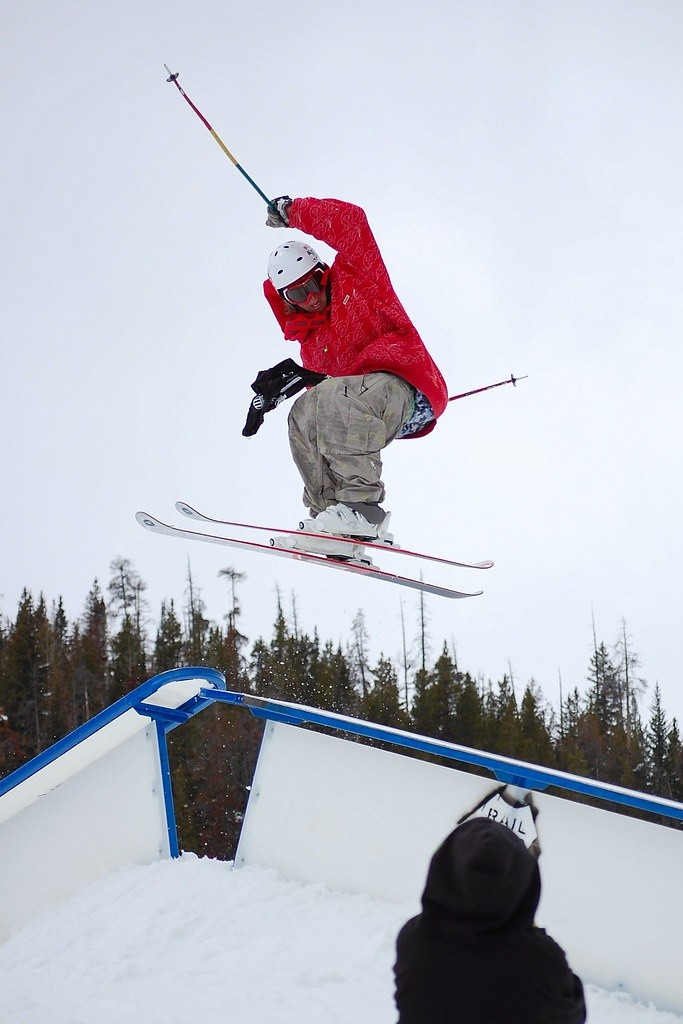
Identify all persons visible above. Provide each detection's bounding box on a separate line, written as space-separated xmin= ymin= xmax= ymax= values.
xmin=391 ymin=817 xmax=587 ymax=1024
xmin=241 ymin=193 xmax=450 ymax=574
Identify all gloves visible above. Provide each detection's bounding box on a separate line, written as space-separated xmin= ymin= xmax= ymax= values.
xmin=265 ymin=196 xmax=292 ymax=228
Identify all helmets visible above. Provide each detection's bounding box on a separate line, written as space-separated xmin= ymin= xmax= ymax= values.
xmin=267 ymin=241 xmax=327 ymax=298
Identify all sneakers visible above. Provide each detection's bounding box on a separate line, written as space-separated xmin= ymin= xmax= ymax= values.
xmin=291 ymin=535 xmax=364 ymax=559
xmin=316 ymin=503 xmax=378 ymax=539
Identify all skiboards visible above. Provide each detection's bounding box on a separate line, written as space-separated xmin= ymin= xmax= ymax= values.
xmin=133 ymin=501 xmax=494 ymax=599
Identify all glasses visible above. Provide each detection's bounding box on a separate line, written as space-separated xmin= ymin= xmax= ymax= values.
xmin=282 ymin=267 xmax=328 ymax=305
